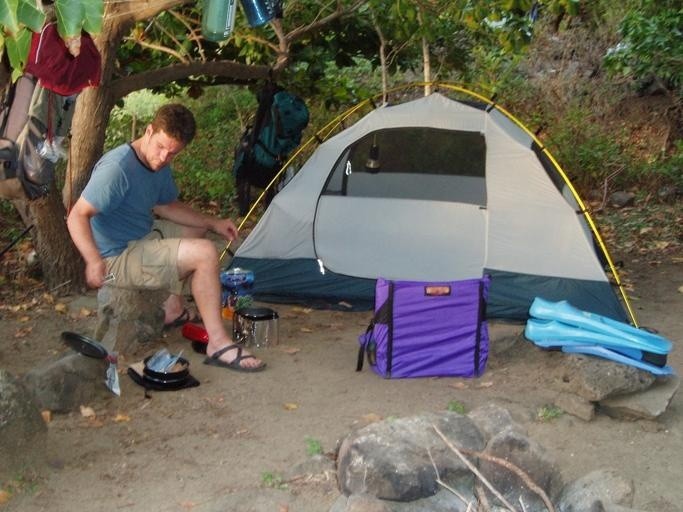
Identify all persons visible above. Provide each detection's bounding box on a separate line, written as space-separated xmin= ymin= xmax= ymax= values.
xmin=67 ymin=104 xmax=268 ymax=373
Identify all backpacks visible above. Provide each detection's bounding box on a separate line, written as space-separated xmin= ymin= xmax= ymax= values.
xmin=230 ymin=78 xmax=311 ymax=194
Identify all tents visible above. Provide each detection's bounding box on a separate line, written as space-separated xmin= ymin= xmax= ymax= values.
xmin=220 ymin=82 xmax=640 ymax=333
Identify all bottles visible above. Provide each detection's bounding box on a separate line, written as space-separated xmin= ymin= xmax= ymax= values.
xmin=240 ymin=0 xmax=274 ymax=27
xmin=200 ymin=0 xmax=236 ymax=41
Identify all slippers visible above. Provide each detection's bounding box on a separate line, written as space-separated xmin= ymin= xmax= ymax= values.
xmin=204 ymin=340 xmax=267 ymax=373
xmin=164 ymin=307 xmax=202 ymax=328
